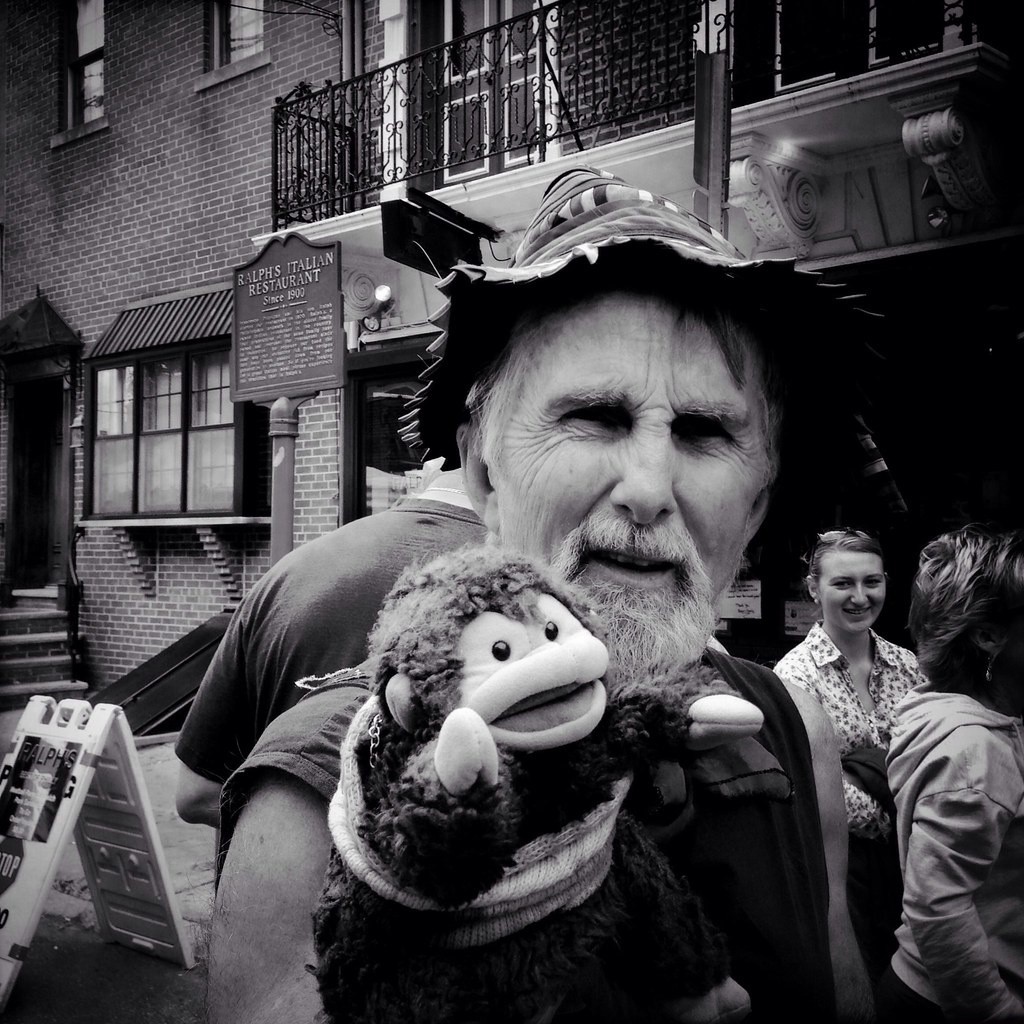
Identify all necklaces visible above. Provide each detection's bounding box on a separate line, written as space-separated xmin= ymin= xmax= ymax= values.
xmin=423 ymin=487 xmax=466 ymax=493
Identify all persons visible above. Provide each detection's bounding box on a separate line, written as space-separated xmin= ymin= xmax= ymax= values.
xmin=171 ymin=468 xmax=487 ymax=826
xmin=772 ymin=527 xmax=918 ymax=976
xmin=206 ymin=165 xmax=889 ymax=1023
xmin=874 ymin=522 xmax=1024 ymax=1024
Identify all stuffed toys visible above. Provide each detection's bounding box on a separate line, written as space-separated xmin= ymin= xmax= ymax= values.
xmin=306 ymin=539 xmax=765 ymax=1024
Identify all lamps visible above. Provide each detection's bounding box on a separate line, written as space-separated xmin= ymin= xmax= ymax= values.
xmin=920 ymin=176 xmax=966 ymax=231
xmin=69 ymin=416 xmax=84 ymax=447
xmin=361 ymin=285 xmax=394 ymax=333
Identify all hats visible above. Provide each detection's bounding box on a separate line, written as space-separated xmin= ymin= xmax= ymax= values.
xmin=397 ymin=166 xmax=890 ymax=543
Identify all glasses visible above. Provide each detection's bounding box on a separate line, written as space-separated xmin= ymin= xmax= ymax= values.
xmin=809 ymin=526 xmax=872 ymax=571
